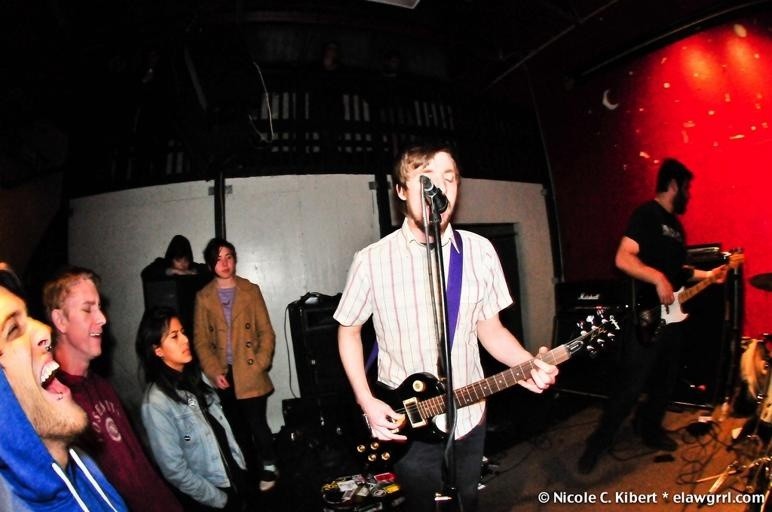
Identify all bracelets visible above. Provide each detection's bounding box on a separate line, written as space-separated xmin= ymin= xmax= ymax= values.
xmin=707 ymin=271 xmax=713 ymax=280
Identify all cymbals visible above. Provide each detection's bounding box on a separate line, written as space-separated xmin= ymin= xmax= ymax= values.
xmin=751 ymin=272 xmax=772 ymax=291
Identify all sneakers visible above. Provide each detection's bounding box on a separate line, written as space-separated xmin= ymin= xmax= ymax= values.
xmin=260 ymin=465 xmax=277 ymax=490
xmin=631 ymin=421 xmax=681 ymax=451
xmin=579 ymin=434 xmax=609 ymax=475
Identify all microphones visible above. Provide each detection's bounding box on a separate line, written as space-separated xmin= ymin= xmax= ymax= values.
xmin=418 ymin=175 xmax=453 ymax=216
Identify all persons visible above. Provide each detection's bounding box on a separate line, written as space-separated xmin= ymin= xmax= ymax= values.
xmin=41 ymin=268 xmax=181 ymax=512
xmin=330 ymin=142 xmax=561 ymax=510
xmin=139 ymin=239 xmax=206 ymax=280
xmin=135 ymin=305 xmax=251 ymax=512
xmin=577 ymin=155 xmax=728 ymax=476
xmin=192 ymin=237 xmax=277 ymax=463
xmin=1 ymin=262 xmax=130 ymax=512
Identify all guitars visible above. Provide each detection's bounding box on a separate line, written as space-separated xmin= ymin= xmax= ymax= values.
xmin=636 ymin=251 xmax=744 ymax=347
xmin=343 ymin=301 xmax=642 ymax=471
